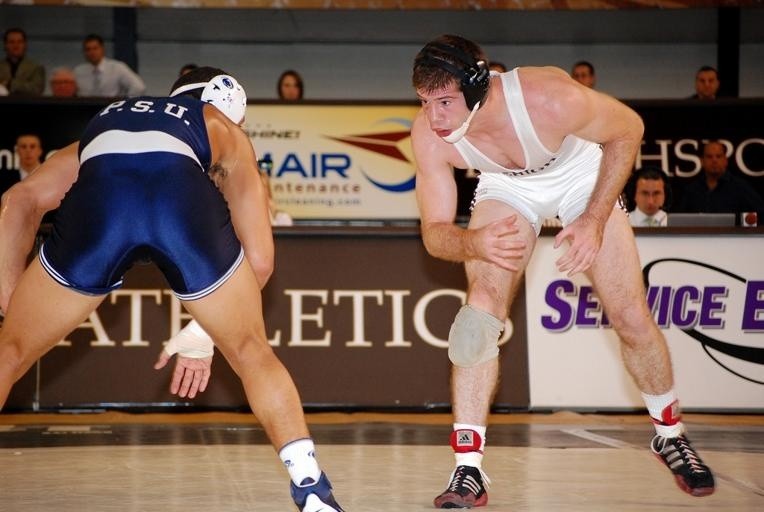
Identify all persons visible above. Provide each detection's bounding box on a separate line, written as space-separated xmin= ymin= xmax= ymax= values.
xmin=0 ymin=28 xmax=45 ymax=97
xmin=625 ymin=168 xmax=667 ymax=228
xmin=487 ymin=60 xmax=507 ymax=74
xmin=0 ymin=66 xmax=346 ymax=511
xmin=689 ymin=62 xmax=721 ymax=103
xmin=1 ymin=126 xmax=43 ymax=193
xmin=409 ymin=37 xmax=715 ymax=510
xmin=276 ymin=68 xmax=304 ymax=101
xmin=685 ymin=142 xmax=762 ymax=228
xmin=70 ymin=35 xmax=146 ymax=99
xmin=571 ymin=58 xmax=598 ymax=92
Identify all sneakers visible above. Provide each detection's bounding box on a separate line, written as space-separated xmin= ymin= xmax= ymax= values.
xmin=433 ymin=467 xmax=490 ymax=508
xmin=652 ymin=431 xmax=715 ymax=495
xmin=291 ymin=470 xmax=345 ymax=512
